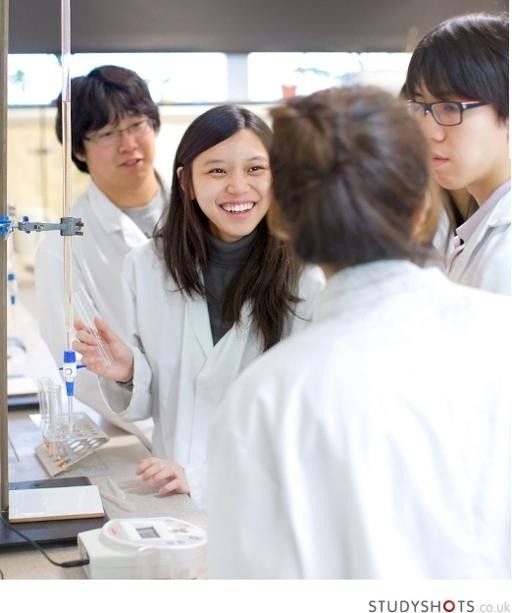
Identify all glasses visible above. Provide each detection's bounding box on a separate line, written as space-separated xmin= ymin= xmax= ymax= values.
xmin=85 ymin=118 xmax=153 ymax=146
xmin=405 ymin=97 xmax=491 ymax=127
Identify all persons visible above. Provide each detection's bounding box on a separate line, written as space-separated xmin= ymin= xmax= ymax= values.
xmin=74 ymin=105 xmax=325 ymax=499
xmin=204 ymin=85 xmax=508 ymax=580
xmin=399 ymin=82 xmax=480 ymax=273
xmin=36 ymin=65 xmax=171 ymax=449
xmin=406 ymin=13 xmax=507 ymax=294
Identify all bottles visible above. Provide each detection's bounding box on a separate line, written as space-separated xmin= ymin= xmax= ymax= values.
xmin=71 ymin=285 xmax=114 ymax=371
xmin=36 ymin=375 xmax=66 ymax=461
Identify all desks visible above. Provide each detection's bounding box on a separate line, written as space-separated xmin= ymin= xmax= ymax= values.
xmin=0 ymin=396 xmax=207 ymax=579
